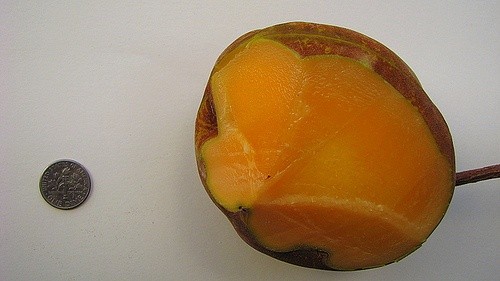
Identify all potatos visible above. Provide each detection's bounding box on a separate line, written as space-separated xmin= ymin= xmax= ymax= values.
xmin=194 ymin=23 xmax=457 ymax=271
